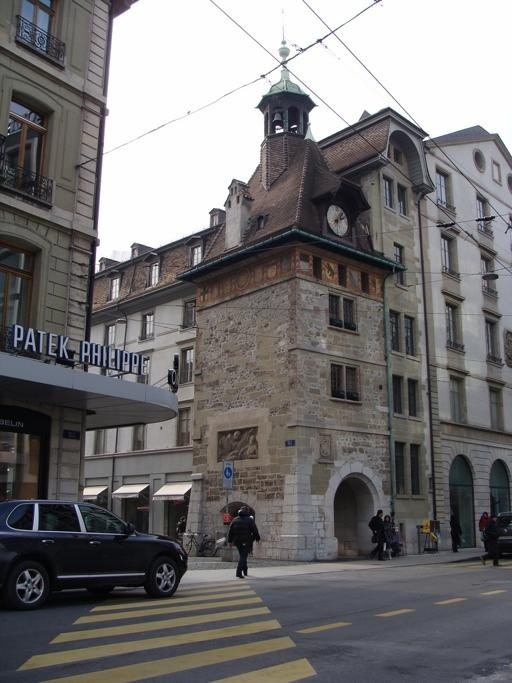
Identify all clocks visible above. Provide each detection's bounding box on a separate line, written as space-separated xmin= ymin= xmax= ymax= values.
xmin=326 ymin=204 xmax=349 ymax=237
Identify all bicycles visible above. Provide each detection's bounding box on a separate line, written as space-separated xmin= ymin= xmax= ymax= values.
xmin=186 ymin=528 xmax=219 ymax=557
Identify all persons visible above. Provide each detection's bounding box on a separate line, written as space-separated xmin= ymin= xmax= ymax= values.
xmin=481 ymin=516 xmax=502 ymax=566
xmin=176 ymin=516 xmax=185 ymax=532
xmin=369 ymin=510 xmax=386 ymax=561
xmin=220 ymin=431 xmax=249 ymax=453
xmin=221 ymin=435 xmax=258 ymax=461
xmin=228 ymin=506 xmax=260 ymax=578
xmin=450 ymin=515 xmax=462 ymax=552
xmin=381 ymin=515 xmax=393 ymax=559
xmin=479 ymin=512 xmax=488 ymax=552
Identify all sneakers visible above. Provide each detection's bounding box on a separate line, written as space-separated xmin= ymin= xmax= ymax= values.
xmin=236 ymin=569 xmax=247 ymax=579
xmin=479 ymin=555 xmax=486 ymax=566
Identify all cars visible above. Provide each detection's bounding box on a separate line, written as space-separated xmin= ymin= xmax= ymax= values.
xmin=496 ymin=512 xmax=512 ymax=556
xmin=0 ymin=497 xmax=189 ymax=609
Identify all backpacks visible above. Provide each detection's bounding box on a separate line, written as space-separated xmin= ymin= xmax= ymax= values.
xmin=231 ymin=519 xmax=252 ymax=547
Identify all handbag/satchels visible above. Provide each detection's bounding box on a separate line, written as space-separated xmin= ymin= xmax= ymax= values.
xmin=390 ymin=540 xmax=401 ymax=554
xmin=372 ymin=531 xmax=387 ymax=543
xmin=480 ymin=531 xmax=489 ymax=541
